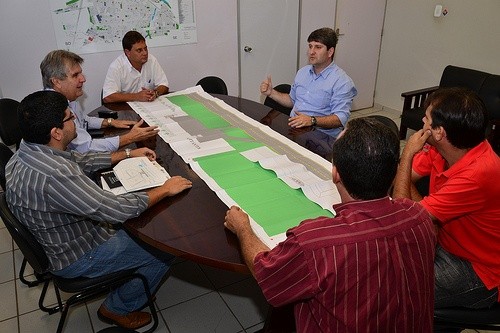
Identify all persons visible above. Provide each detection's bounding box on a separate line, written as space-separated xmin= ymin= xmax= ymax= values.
xmin=224 ymin=118 xmax=437 ymax=333
xmin=391 ymin=90 xmax=500 ymax=310
xmin=39 ymin=50 xmax=160 ymax=154
xmin=101 ymin=31 xmax=169 ymax=106
xmin=260 ymin=28 xmax=357 ymax=138
xmin=5 ymin=90 xmax=193 ymax=330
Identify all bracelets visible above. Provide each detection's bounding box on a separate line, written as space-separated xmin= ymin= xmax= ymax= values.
xmin=125 ymin=148 xmax=132 ymax=158
xmin=107 ymin=118 xmax=114 ymax=126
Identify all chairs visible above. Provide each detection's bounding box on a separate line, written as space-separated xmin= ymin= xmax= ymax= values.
xmin=0 ymin=192 xmax=159 ymax=333
xmin=0 ymin=98 xmax=21 ymax=152
xmin=263 ymin=84 xmax=293 ymax=116
xmin=0 ymin=142 xmax=14 ymax=192
xmin=433 ymin=306 xmax=500 ymax=333
xmin=196 ymin=76 xmax=228 ymax=95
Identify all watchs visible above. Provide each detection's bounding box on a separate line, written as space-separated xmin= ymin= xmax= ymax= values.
xmin=311 ymin=116 xmax=317 ymax=125
xmin=155 ymin=89 xmax=160 ymax=97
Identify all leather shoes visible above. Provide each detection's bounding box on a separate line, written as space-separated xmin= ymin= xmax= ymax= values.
xmin=97 ymin=304 xmax=152 ymax=330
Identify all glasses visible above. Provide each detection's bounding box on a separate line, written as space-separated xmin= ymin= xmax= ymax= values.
xmin=61 ymin=112 xmax=76 ymax=123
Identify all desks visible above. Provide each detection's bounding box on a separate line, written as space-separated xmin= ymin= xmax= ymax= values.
xmin=87 ymin=92 xmax=337 ymax=330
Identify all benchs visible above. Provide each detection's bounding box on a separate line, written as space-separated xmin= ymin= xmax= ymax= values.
xmin=399 ymin=64 xmax=500 ymax=154
